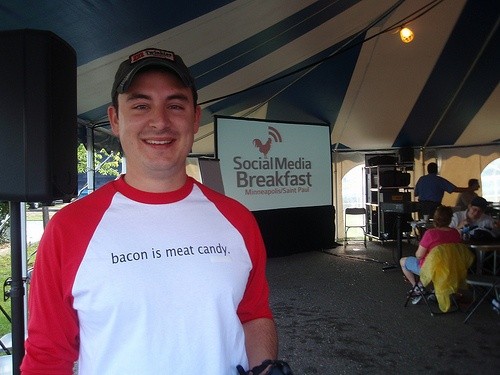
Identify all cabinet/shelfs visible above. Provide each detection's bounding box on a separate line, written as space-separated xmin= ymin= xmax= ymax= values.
xmin=364 ymin=154 xmax=415 ymax=242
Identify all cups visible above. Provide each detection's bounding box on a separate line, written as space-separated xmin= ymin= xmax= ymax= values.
xmin=424 ymin=214 xmax=429 ymax=220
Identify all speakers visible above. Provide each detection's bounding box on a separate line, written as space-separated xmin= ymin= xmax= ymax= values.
xmin=0 ymin=28 xmax=78 ymax=201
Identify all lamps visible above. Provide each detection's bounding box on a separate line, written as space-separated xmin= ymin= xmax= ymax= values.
xmin=400 ymin=25 xmax=415 ymax=43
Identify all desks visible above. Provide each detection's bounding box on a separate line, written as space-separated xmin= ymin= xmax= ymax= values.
xmin=382 ymin=210 xmax=415 ymax=271
xmin=469 ymin=244 xmax=500 ymax=275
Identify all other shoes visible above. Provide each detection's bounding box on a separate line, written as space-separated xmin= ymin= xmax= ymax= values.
xmin=413 ymin=291 xmax=426 ymax=305
xmin=407 ymin=286 xmax=425 ymax=295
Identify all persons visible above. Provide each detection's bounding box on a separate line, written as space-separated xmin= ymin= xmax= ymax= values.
xmin=450 ymin=198 xmax=497 ymax=234
xmin=415 ymin=162 xmax=468 ymax=221
xmin=20 ymin=48 xmax=278 ymax=375
xmin=457 ymin=177 xmax=480 ymax=209
xmin=400 ymin=206 xmax=462 ymax=305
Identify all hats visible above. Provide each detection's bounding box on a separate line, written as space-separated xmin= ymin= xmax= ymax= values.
xmin=471 ymin=196 xmax=488 ymax=211
xmin=111 ymin=48 xmax=197 ymax=106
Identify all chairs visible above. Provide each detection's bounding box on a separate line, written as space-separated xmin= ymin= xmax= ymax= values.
xmin=464 ymin=271 xmax=500 ymax=323
xmin=404 ymin=243 xmax=473 ymax=316
